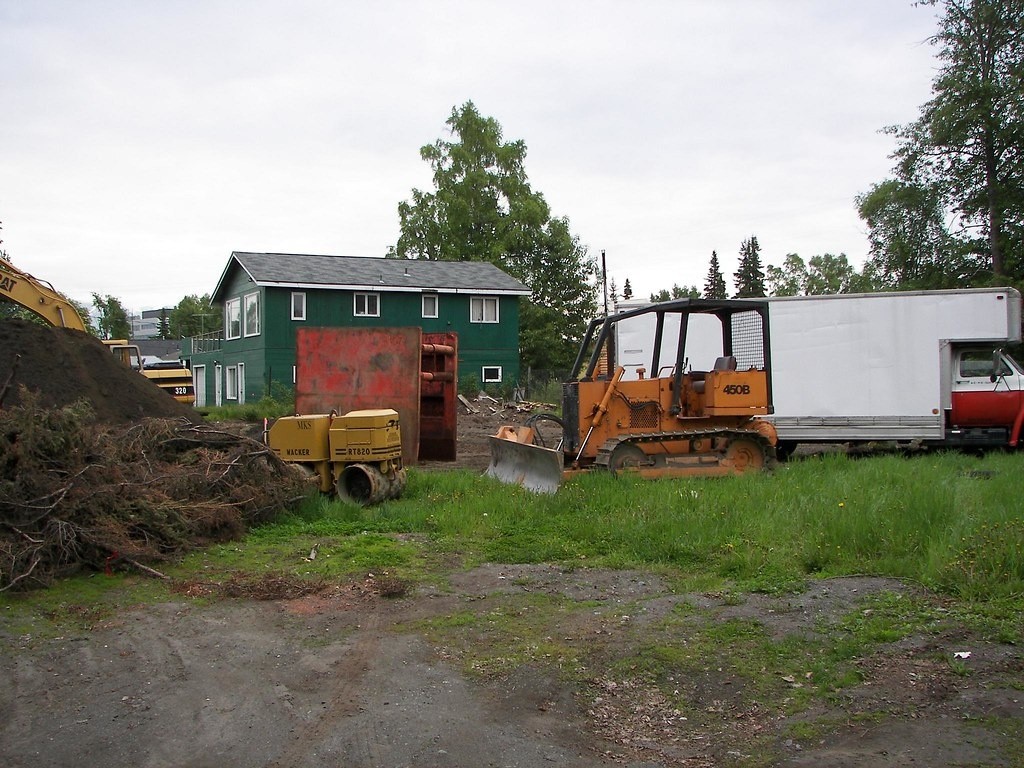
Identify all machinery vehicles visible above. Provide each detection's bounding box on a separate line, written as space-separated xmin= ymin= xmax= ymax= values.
xmin=0 ymin=257 xmax=194 ymax=404
xmin=481 ymin=298 xmax=777 ymax=494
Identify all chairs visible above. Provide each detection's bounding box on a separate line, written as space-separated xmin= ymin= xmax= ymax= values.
xmin=688 ymin=356 xmax=737 ymax=393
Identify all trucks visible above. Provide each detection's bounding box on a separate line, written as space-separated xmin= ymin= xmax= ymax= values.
xmin=613 ymin=286 xmax=1024 ymax=459
